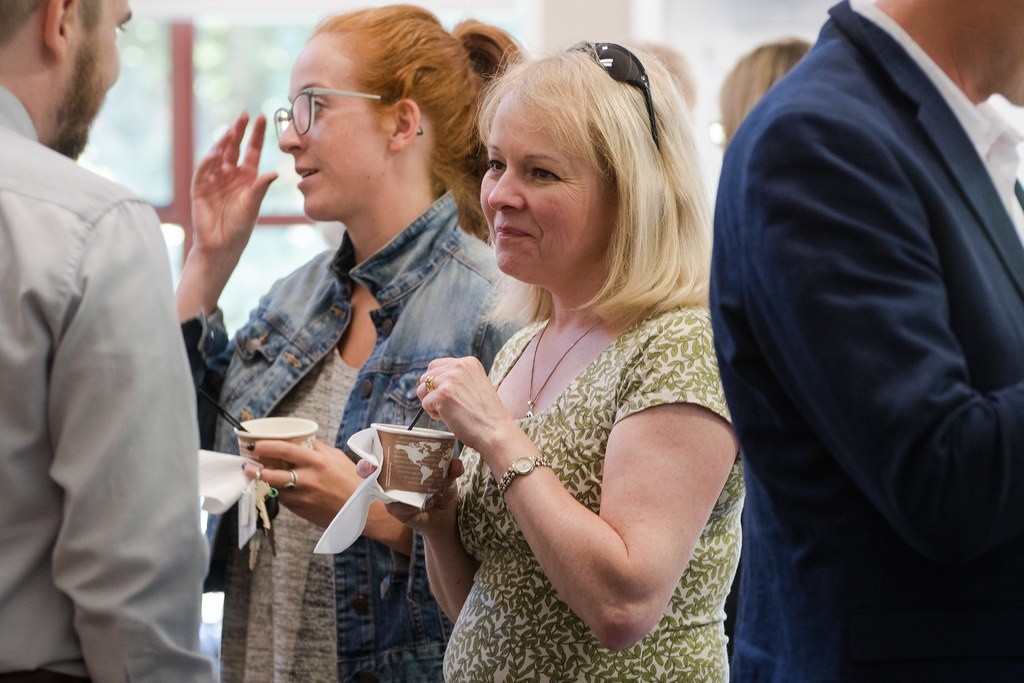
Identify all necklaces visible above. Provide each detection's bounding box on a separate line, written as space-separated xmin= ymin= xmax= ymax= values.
xmin=525 ymin=318 xmax=603 ymax=418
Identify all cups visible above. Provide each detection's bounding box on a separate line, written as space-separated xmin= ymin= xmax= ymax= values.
xmin=235 ymin=417 xmax=317 ymax=472
xmin=368 ymin=422 xmax=455 ymax=495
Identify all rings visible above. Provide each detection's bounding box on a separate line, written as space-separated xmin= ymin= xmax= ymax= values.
xmin=284 ymin=469 xmax=298 ymax=489
xmin=425 ymin=374 xmax=435 ymax=393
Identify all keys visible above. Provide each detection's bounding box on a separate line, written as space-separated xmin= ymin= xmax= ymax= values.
xmin=238 ymin=466 xmax=280 ymax=572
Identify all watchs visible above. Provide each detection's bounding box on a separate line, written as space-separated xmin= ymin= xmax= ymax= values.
xmin=498 ymin=455 xmax=554 ymax=498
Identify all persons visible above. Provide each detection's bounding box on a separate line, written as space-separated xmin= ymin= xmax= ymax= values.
xmin=359 ymin=42 xmax=749 ymax=683
xmin=173 ymin=5 xmax=534 ymax=683
xmin=709 ymin=1 xmax=1023 ymax=683
xmin=0 ymin=0 xmax=219 ymax=683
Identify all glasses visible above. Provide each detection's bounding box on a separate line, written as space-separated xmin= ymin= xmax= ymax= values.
xmin=565 ymin=40 xmax=659 ymax=151
xmin=275 ymin=86 xmax=391 ymax=135
